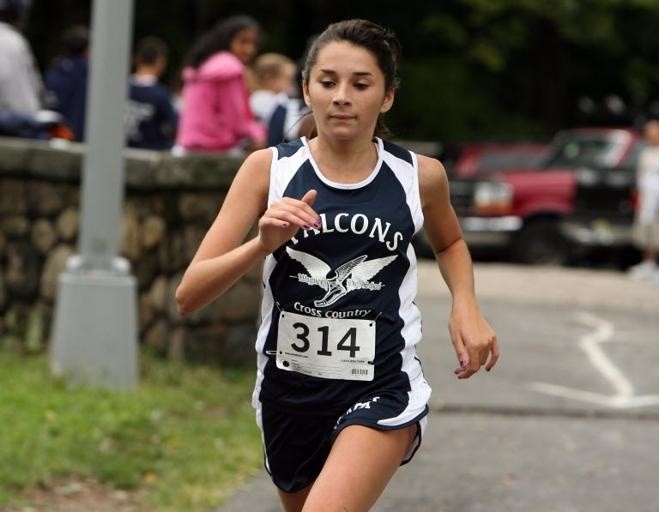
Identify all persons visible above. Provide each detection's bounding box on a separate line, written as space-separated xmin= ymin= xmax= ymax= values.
xmin=0 ymin=14 xmax=314 ymax=156
xmin=626 ymin=119 xmax=659 ymax=277
xmin=174 ymin=18 xmax=500 ymax=512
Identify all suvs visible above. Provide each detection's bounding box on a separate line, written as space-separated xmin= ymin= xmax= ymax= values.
xmin=413 ymin=126 xmax=658 ymax=268
